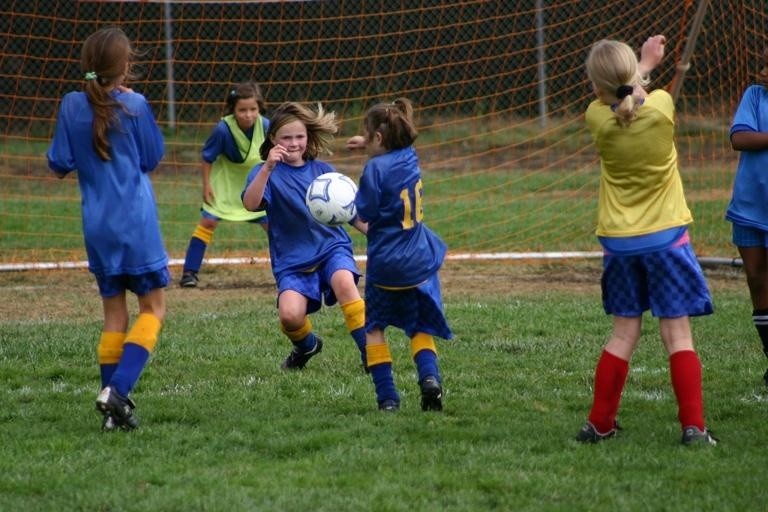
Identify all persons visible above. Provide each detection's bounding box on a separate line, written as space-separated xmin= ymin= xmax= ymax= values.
xmin=574 ymin=34 xmax=716 ymax=448
xmin=176 ymin=80 xmax=271 ymax=288
xmin=47 ymin=26 xmax=172 ymax=431
xmin=243 ymin=99 xmax=371 ymax=377
xmin=342 ymin=96 xmax=454 ymax=415
xmin=725 ymin=46 xmax=768 ymax=390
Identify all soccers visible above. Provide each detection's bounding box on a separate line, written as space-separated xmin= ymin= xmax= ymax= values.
xmin=305 ymin=172 xmax=359 ymax=226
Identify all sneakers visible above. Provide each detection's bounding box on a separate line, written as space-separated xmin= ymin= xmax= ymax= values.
xmin=418 ymin=377 xmax=443 ymax=410
xmin=380 ymin=398 xmax=399 ymax=410
xmin=683 ymin=426 xmax=717 ymax=445
xmin=576 ymin=420 xmax=617 ymax=441
xmin=282 ymin=339 xmax=323 ymax=368
xmin=94 ymin=387 xmax=139 ymax=430
xmin=180 ymin=272 xmax=198 ymax=286
